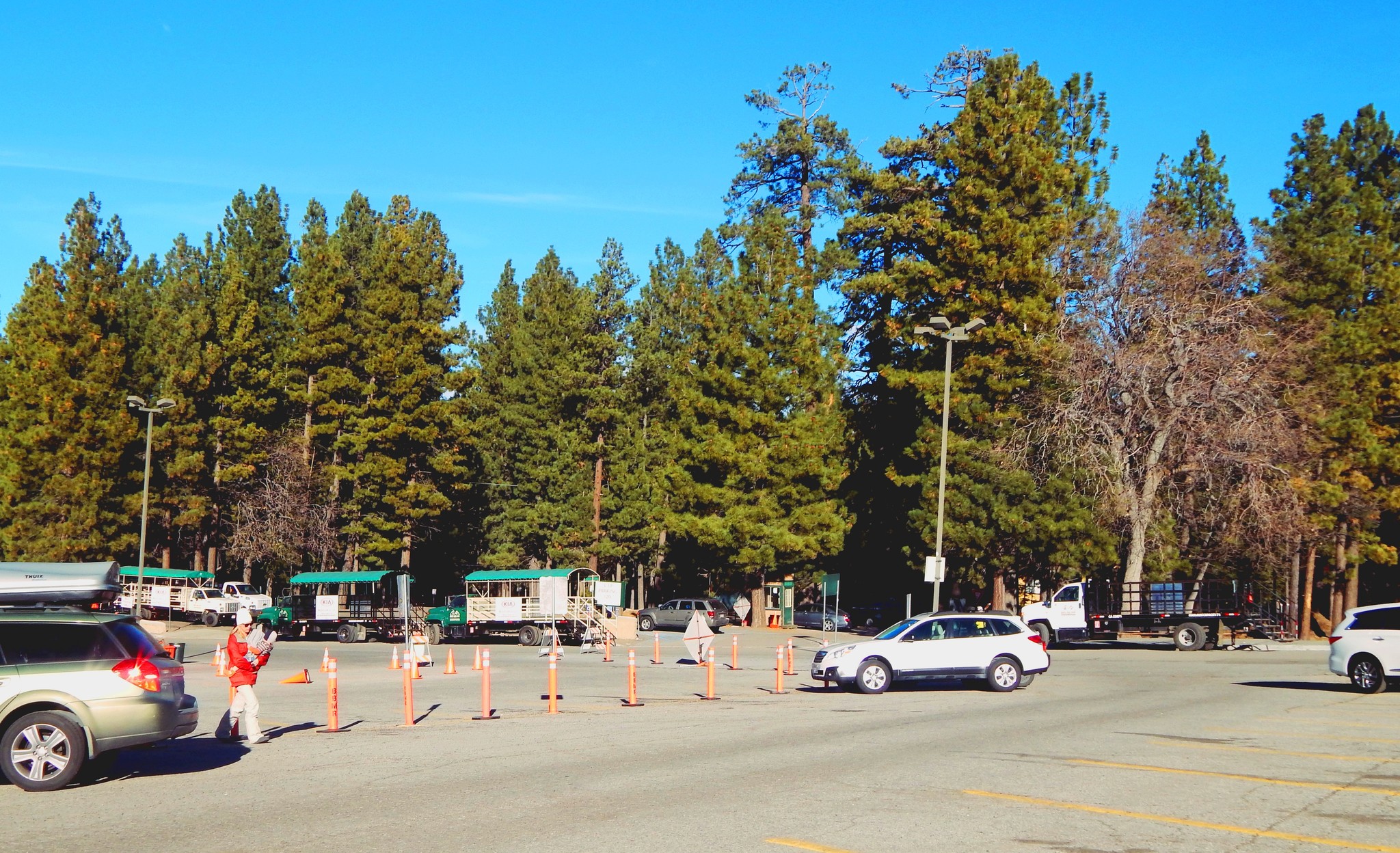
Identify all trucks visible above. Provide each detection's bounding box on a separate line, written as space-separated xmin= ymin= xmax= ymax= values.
xmin=257 ymin=571 xmax=423 ymax=643
xmin=1022 ymin=577 xmax=1253 ymax=651
xmin=425 ymin=568 xmax=601 ymax=647
xmin=114 ymin=566 xmax=272 ymax=627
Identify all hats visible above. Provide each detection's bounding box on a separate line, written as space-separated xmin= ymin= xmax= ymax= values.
xmin=236 ymin=608 xmax=253 ymax=625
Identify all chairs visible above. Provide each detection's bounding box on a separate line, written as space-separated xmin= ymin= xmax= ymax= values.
xmin=812 ymin=605 xmax=822 ymax=613
xmin=930 ymin=623 xmax=947 ymax=639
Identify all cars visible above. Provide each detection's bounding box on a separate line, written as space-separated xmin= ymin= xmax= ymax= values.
xmin=793 ymin=603 xmax=852 ymax=632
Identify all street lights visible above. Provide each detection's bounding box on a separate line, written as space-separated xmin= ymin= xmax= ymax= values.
xmin=125 ymin=395 xmax=175 ymax=625
xmin=913 ymin=316 xmax=988 ymax=638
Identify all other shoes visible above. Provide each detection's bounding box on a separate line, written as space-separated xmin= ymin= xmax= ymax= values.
xmin=224 ymin=667 xmax=236 ymax=677
xmin=215 ymin=735 xmax=234 ymax=744
xmin=253 ymin=736 xmax=269 ymax=743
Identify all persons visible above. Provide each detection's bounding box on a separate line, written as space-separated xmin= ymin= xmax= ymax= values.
xmin=215 ymin=609 xmax=271 ymax=743
xmin=974 ymin=606 xmax=986 ymax=629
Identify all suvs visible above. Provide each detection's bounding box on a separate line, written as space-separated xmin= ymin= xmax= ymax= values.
xmin=811 ymin=611 xmax=1050 ymax=694
xmin=1 ymin=561 xmax=199 ymax=793
xmin=1328 ymin=603 xmax=1400 ymax=694
xmin=637 ymin=598 xmax=729 ymax=633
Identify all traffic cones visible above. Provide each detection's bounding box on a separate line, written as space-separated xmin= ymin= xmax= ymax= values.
xmin=442 ymin=648 xmax=457 ymax=674
xmin=216 ymin=650 xmax=227 ymax=676
xmin=211 ymin=643 xmax=221 ymax=665
xmin=318 ymin=647 xmax=329 ymax=672
xmin=472 ymin=646 xmax=483 ymax=670
xmin=410 ymin=652 xmax=422 ymax=679
xmin=770 ymin=611 xmax=779 ymax=628
xmin=387 ymin=646 xmax=402 ymax=669
xmin=278 ymin=669 xmax=311 ymax=683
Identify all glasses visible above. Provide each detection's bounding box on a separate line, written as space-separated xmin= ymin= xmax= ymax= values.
xmin=242 ymin=621 xmax=254 ymax=627
